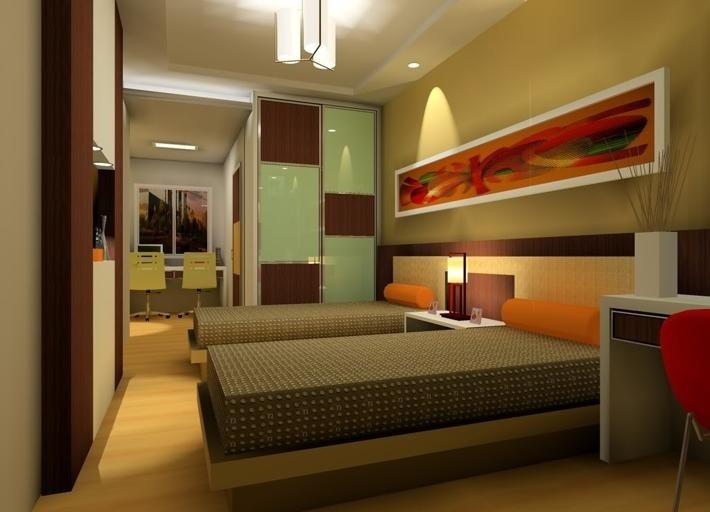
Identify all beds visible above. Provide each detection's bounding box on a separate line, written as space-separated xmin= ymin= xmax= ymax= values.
xmin=188 ymin=279 xmax=436 ymax=384
xmin=196 ymin=299 xmax=605 ymax=510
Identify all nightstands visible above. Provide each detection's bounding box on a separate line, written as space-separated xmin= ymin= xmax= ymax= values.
xmin=403 ymin=308 xmax=505 ymax=332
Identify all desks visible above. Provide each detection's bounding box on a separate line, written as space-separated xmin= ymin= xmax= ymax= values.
xmin=589 ymin=289 xmax=710 ymax=465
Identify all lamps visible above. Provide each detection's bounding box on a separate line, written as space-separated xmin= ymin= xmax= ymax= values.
xmin=269 ymin=0 xmax=338 ymax=70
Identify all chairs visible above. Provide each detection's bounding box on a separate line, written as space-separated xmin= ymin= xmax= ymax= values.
xmin=129 ymin=250 xmax=218 ymax=321
xmin=658 ymin=304 xmax=710 ymax=512
